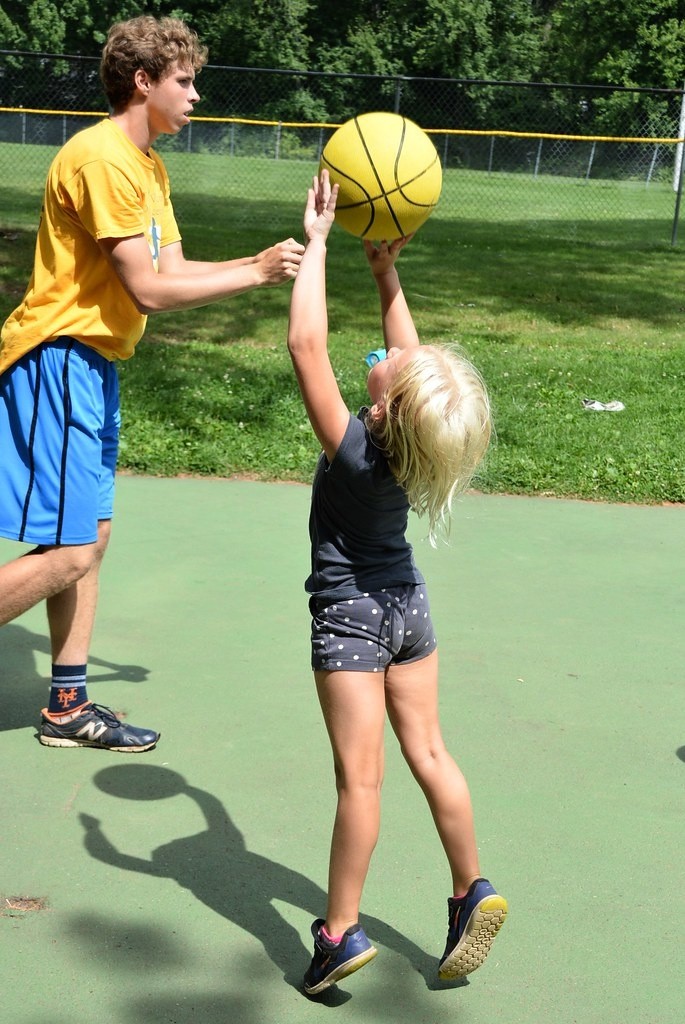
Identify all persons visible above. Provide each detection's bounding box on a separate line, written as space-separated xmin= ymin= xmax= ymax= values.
xmin=0 ymin=16 xmax=307 ymax=753
xmin=284 ymin=168 xmax=510 ymax=997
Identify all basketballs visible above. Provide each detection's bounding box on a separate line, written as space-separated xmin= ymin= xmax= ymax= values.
xmin=318 ymin=112 xmax=442 ymax=238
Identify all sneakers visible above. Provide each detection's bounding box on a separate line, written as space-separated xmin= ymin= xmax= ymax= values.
xmin=304 ymin=918 xmax=377 ymax=994
xmin=438 ymin=877 xmax=508 ymax=981
xmin=40 ymin=699 xmax=161 ymax=752
